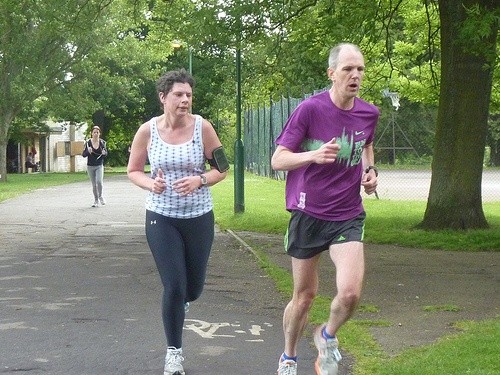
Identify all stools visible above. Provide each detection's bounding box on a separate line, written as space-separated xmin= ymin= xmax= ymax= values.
xmin=27 ymin=168 xmax=32 ymax=174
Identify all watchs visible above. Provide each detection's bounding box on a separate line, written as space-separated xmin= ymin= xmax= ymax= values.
xmin=197 ymin=174 xmax=207 ymax=189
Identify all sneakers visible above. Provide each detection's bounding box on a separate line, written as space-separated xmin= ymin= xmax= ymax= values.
xmin=163 ymin=346 xmax=185 ymax=375
xmin=277 ymin=353 xmax=298 ymax=375
xmin=313 ymin=322 xmax=342 ymax=375
xmin=184 ymin=302 xmax=190 ymax=314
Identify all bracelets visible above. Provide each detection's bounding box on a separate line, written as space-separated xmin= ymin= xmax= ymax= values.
xmin=365 ymin=166 xmax=378 ymax=177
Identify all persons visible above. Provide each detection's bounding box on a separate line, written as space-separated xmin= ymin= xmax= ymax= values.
xmin=82 ymin=126 xmax=108 ymax=207
xmin=127 ymin=68 xmax=232 ymax=375
xmin=26 ymin=152 xmax=39 ymax=171
xmin=270 ymin=44 xmax=380 ymax=375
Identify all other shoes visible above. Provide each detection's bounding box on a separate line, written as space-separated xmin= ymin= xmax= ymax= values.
xmin=92 ymin=202 xmax=100 ymax=207
xmin=99 ymin=196 xmax=105 ymax=205
